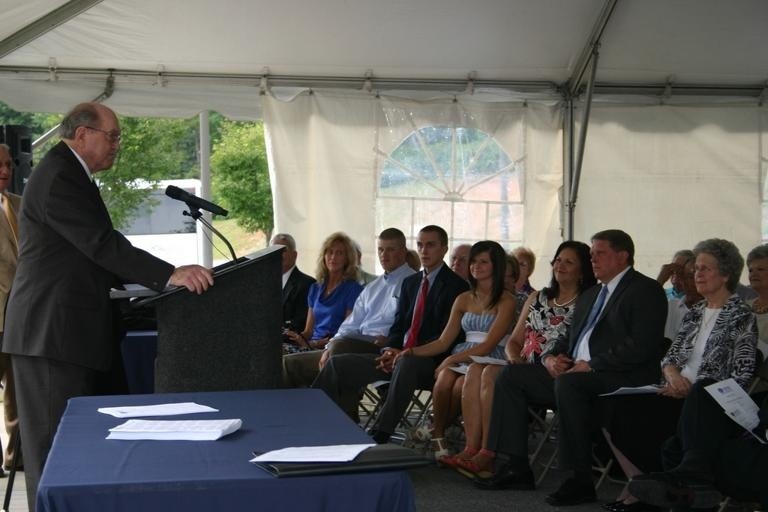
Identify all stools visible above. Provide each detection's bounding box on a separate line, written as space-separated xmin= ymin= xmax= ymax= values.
xmin=347 ymin=371 xmax=767 ymax=512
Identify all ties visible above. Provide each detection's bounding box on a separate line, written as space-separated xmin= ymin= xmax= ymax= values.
xmin=403 ymin=277 xmax=429 ymax=351
xmin=2 ymin=192 xmax=19 ymax=249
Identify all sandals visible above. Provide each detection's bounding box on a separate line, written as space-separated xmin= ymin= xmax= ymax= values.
xmin=416 ymin=423 xmax=496 ymax=471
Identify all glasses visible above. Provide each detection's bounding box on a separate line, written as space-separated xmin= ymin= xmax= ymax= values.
xmin=85 ymin=126 xmax=121 ymax=142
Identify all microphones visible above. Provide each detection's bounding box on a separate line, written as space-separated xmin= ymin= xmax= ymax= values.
xmin=165 ymin=184 xmax=229 ymax=217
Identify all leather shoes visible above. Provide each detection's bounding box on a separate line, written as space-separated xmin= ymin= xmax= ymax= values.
xmin=472 ymin=471 xmax=692 ymax=511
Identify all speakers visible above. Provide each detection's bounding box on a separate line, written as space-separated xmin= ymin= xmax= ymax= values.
xmin=1 ymin=124 xmax=34 ymax=195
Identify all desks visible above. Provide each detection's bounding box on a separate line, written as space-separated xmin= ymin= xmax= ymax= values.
xmin=34 ymin=388 xmax=416 ymax=512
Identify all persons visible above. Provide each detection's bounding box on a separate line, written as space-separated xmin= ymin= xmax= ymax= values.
xmin=0 ymin=102 xmax=215 ymax=512
xmin=274 ymin=225 xmax=768 ymax=511
xmin=0 ymin=142 xmax=22 ymax=478
